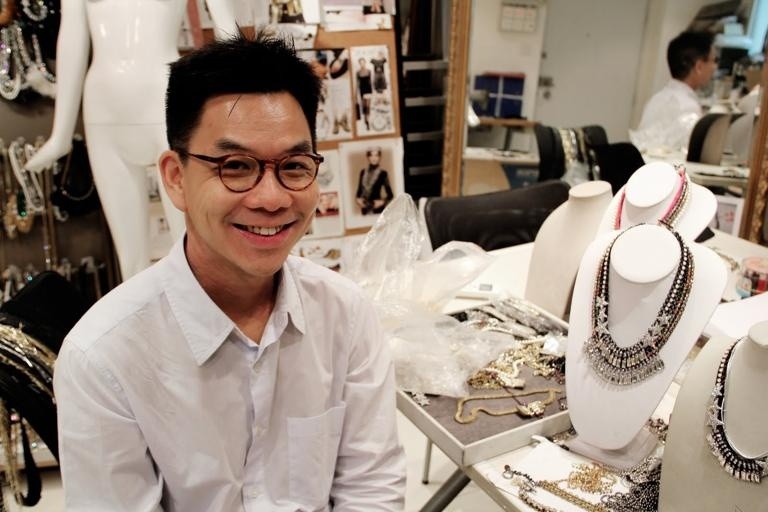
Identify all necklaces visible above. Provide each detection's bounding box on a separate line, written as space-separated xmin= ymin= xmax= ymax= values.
xmin=0 ymin=311 xmax=59 ymax=512
xmin=451 ymin=342 xmax=670 ymax=512
xmin=614 ymin=164 xmax=691 ymax=232
xmin=0 ymin=0 xmax=96 ymax=240
xmin=581 ymin=221 xmax=696 ymax=386
xmin=703 ymin=336 xmax=768 ymax=485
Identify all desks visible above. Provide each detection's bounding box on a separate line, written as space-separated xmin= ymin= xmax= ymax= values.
xmin=415 ymin=230 xmax=768 ymax=512
xmin=464 ymin=147 xmax=748 ymax=190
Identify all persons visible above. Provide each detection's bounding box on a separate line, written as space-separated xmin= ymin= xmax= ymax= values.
xmin=370 ymin=51 xmax=387 ymax=91
xmin=53 ymin=34 xmax=411 ymax=512
xmin=356 ymin=145 xmax=394 ymax=216
xmin=637 ymin=29 xmax=718 ymax=160
xmin=354 ymin=57 xmax=372 ymax=131
xmin=22 ymin=0 xmax=242 ymax=283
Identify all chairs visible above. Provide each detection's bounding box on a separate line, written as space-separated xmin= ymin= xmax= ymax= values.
xmin=421 ymin=180 xmax=572 ymax=483
xmin=587 ymin=140 xmax=644 ymax=196
xmin=534 ymin=125 xmax=608 ymax=182
xmin=0 ymin=271 xmax=93 ymax=468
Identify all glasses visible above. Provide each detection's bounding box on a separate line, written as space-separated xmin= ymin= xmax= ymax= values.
xmin=183 ymin=150 xmax=324 ymax=193
